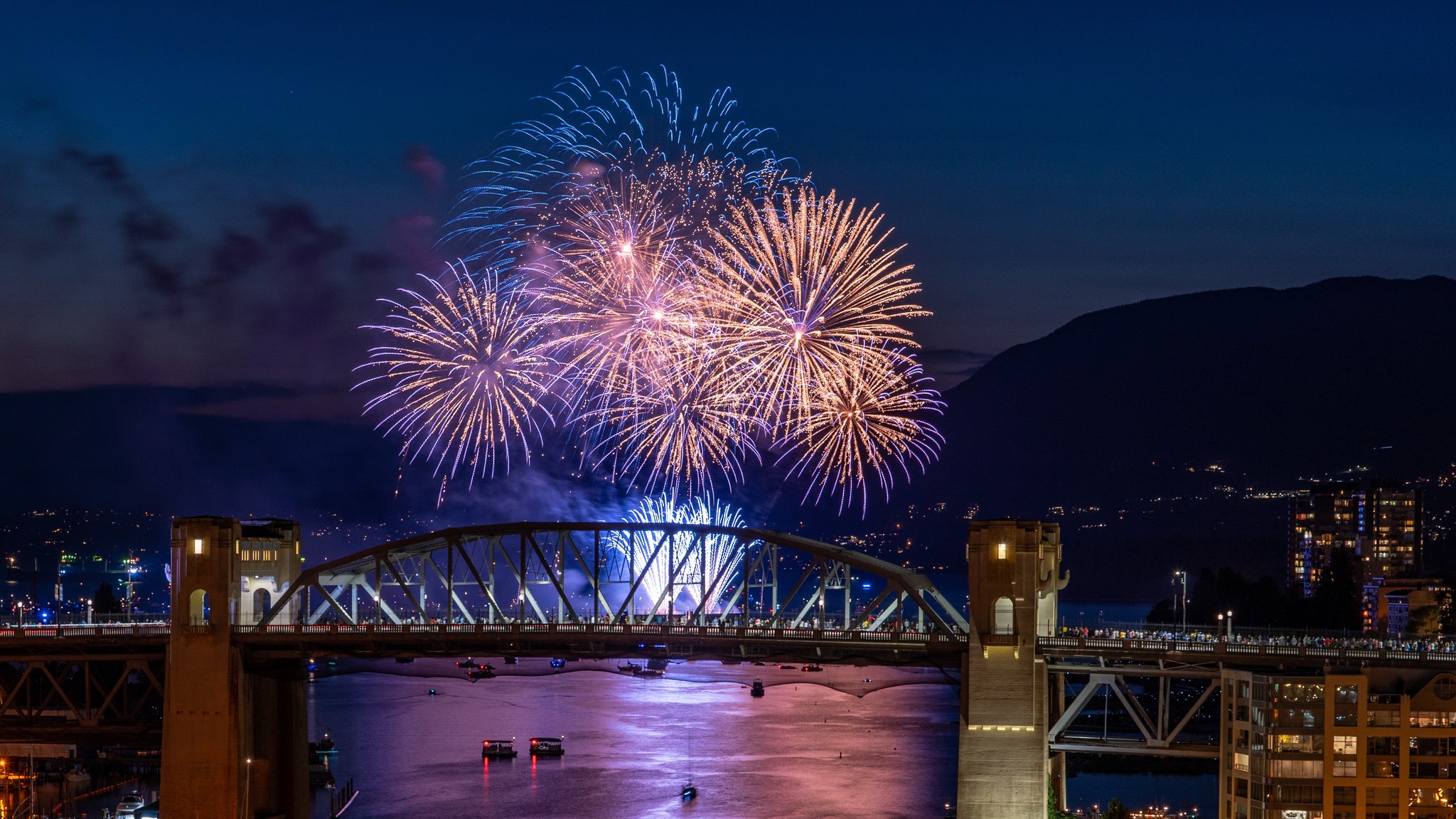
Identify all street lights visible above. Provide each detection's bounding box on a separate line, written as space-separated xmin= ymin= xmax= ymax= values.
xmin=122 ymin=559 xmax=136 ymax=624
xmin=1176 ymin=571 xmax=1186 ymax=632
xmin=1218 ymin=614 xmax=1223 ymax=642
xmin=57 ymin=555 xmax=76 ymax=637
xmin=1227 ymin=610 xmax=1232 ymax=643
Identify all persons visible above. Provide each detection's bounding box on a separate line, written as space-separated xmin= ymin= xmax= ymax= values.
xmin=299 ymin=614 xmax=957 ymax=634
xmin=0 ymin=618 xmax=171 ymax=629
xmin=1055 ymin=626 xmax=1455 ymax=652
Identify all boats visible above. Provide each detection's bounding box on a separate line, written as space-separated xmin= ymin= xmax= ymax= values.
xmin=137 ymin=746 xmax=160 ymax=757
xmin=780 ymin=665 xmax=798 ymax=669
xmin=750 ymin=678 xmax=765 ymax=697
xmin=721 ymin=660 xmax=741 ymax=665
xmin=550 ymin=657 xmax=567 ymax=667
xmin=682 ymin=787 xmax=696 ymax=798
xmin=754 ymin=662 xmax=765 ymax=666
xmin=801 ymin=664 xmax=823 ymax=672
xmin=66 ymin=768 xmax=90 ymax=782
xmin=466 ymin=664 xmax=496 ymax=679
xmin=481 ymin=739 xmax=518 ymax=760
xmin=395 ymin=656 xmax=414 ymax=663
xmin=308 ymin=742 xmax=336 ymax=789
xmin=317 ymin=738 xmax=335 ymax=752
xmin=113 ymin=788 xmax=144 ymax=819
xmin=0 ymin=775 xmax=36 ymax=789
xmin=504 ymin=656 xmax=519 ymax=664
xmin=646 ymin=659 xmax=668 ymax=670
xmin=529 ymin=737 xmax=565 ymax=755
xmin=456 ymin=657 xmax=478 ymax=668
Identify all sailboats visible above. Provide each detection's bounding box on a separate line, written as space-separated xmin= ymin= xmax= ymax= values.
xmin=631 ymin=656 xmax=665 ymax=677
xmin=617 ymin=656 xmax=642 ymax=672
